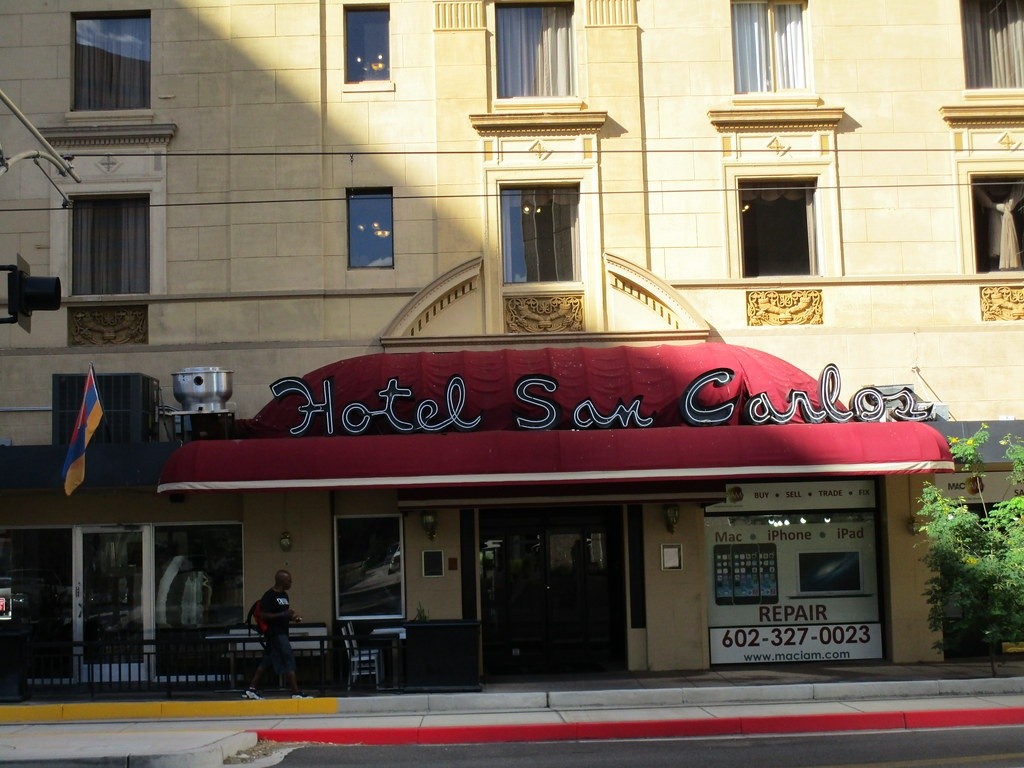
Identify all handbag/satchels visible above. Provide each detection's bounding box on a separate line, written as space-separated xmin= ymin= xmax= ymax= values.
xmin=244 ymin=599 xmax=271 ymax=636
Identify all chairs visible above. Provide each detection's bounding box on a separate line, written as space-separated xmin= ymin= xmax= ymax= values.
xmin=340 ymin=620 xmax=384 ymax=694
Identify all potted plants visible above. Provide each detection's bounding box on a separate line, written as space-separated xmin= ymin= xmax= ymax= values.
xmin=405 ymin=607 xmax=483 ymax=694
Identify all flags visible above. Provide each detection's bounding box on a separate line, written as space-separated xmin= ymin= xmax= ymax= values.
xmin=61 ymin=369 xmax=107 ymax=498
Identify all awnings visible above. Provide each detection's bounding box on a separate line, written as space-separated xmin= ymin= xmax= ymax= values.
xmin=152 ymin=419 xmax=953 ymax=474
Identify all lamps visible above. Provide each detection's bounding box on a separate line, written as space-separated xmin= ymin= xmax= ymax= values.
xmin=907 ymin=517 xmax=922 ymax=536
xmin=278 ymin=530 xmax=293 ymax=553
xmin=661 ymin=503 xmax=681 ymax=533
xmin=420 ymin=509 xmax=438 ymax=540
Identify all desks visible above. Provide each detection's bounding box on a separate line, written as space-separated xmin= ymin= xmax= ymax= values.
xmin=370 ymin=625 xmax=406 ymax=691
xmin=204 ymin=633 xmax=308 ymax=691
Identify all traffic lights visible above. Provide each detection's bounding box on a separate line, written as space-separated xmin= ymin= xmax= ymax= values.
xmin=7 ymin=251 xmax=63 ymax=335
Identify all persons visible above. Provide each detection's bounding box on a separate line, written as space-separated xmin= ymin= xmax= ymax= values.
xmin=241 ymin=569 xmax=315 ymax=698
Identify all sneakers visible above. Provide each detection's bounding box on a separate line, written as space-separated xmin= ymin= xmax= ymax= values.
xmin=240 ymin=687 xmax=266 ymax=700
xmin=291 ymin=692 xmax=314 ymax=698
